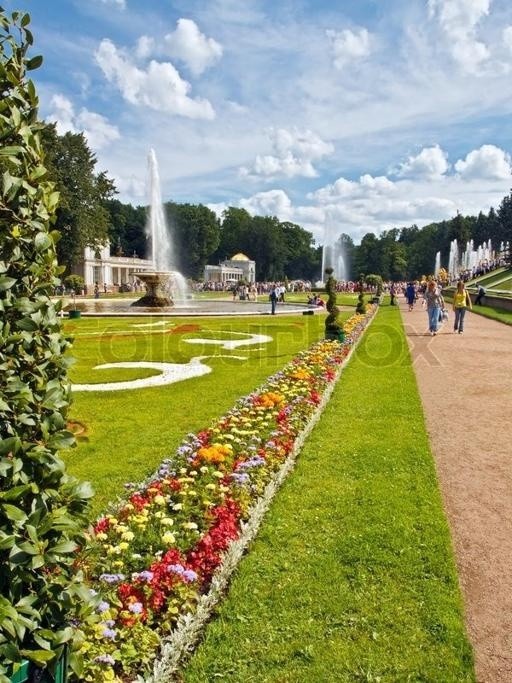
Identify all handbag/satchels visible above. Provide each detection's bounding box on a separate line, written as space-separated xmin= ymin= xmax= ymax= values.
xmin=440 ymin=309 xmax=448 ymax=321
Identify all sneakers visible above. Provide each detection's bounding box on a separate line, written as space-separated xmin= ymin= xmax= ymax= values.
xmin=429 ymin=329 xmax=437 ymax=335
xmin=454 ymin=328 xmax=464 ymax=334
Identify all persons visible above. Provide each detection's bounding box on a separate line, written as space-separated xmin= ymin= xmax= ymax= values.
xmin=405 ymin=282 xmax=415 ymax=312
xmin=425 ymin=280 xmax=445 ymax=336
xmin=474 ymin=284 xmax=486 ymax=305
xmin=390 ymin=284 xmax=396 ymax=305
xmin=94 ymin=281 xmax=99 ymax=298
xmin=335 ymin=257 xmax=499 ymax=294
xmin=453 ymin=281 xmax=472 ymax=335
xmin=195 ymin=280 xmax=326 ymax=315
xmin=104 ymin=283 xmax=107 ymax=292
